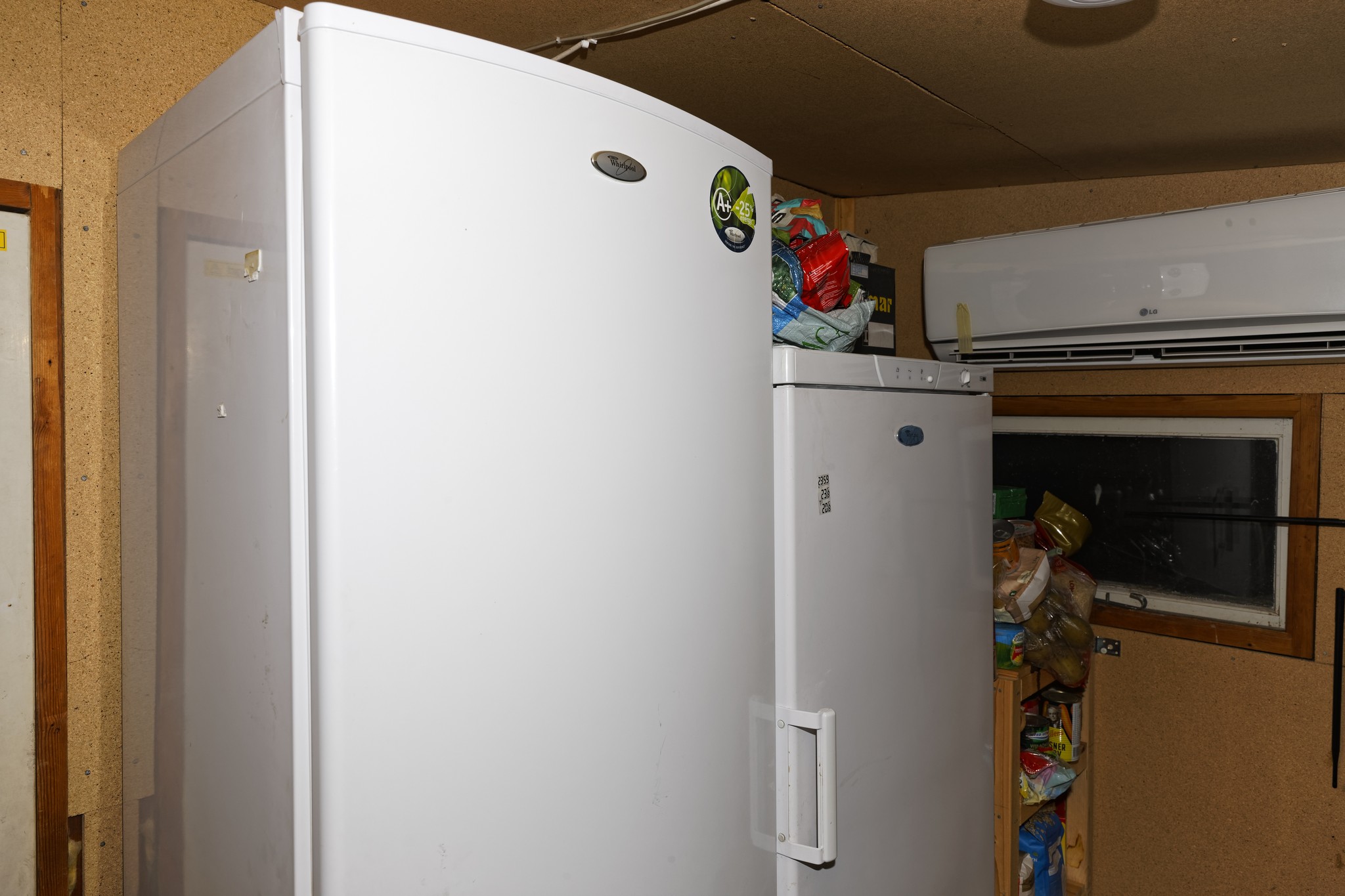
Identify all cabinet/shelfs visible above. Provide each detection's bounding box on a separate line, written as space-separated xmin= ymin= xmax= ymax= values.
xmin=994 ymin=650 xmax=1093 ymax=896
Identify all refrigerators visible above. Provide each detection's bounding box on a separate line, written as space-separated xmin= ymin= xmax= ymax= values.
xmin=773 ymin=344 xmax=994 ymax=896
xmin=119 ymin=2 xmax=776 ymax=896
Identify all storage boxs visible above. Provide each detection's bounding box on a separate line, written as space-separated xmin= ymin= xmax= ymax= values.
xmin=849 ymin=260 xmax=896 ymax=357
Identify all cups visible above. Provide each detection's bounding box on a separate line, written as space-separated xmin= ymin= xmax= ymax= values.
xmin=1007 ymin=519 xmax=1037 ymax=548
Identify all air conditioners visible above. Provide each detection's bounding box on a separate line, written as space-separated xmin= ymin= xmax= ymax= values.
xmin=923 ymin=186 xmax=1345 ymax=371
